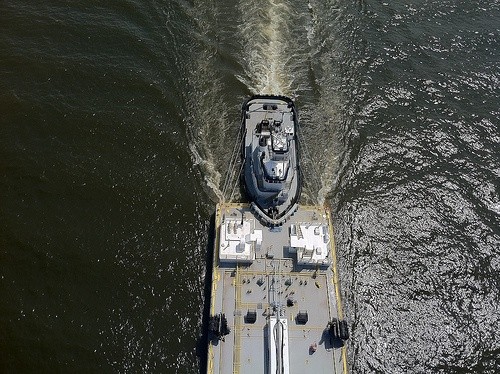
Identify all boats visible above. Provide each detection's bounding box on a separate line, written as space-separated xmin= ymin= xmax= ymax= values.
xmin=207 ymin=203 xmax=349 ymax=374
xmin=241 ymin=94 xmax=301 ymax=202
